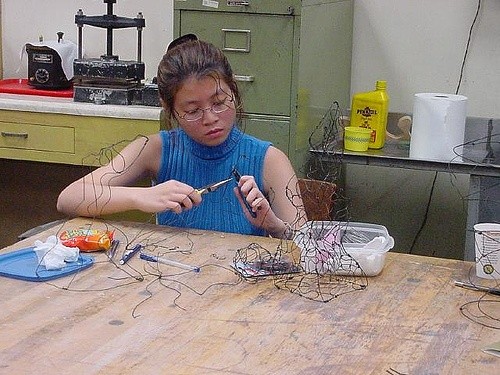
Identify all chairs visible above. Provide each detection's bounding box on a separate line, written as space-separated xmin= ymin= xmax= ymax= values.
xmin=297 ymin=180 xmax=337 ymax=222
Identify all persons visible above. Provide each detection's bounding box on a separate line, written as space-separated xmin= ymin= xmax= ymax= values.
xmin=57 ymin=42 xmax=306 ymax=240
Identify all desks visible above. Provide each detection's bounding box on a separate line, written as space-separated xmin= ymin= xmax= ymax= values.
xmin=0 ymin=93 xmax=163 ymax=167
xmin=0 ymin=217 xmax=500 ymax=375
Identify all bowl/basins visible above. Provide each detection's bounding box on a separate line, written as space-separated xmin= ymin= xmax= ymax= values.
xmin=344 ymin=126 xmax=372 ymax=151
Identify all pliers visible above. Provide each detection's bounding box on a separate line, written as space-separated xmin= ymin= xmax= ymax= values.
xmin=231 ymin=165 xmax=262 ymax=218
xmin=170 ymin=178 xmax=234 ymax=210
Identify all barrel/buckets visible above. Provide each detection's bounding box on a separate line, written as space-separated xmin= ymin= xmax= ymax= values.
xmin=474 ymin=222 xmax=500 ymax=280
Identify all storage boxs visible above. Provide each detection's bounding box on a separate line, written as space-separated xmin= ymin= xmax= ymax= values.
xmin=293 ymin=221 xmax=394 ymax=276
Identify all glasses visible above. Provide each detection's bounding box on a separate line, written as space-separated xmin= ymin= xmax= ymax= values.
xmin=173 ymin=90 xmax=235 ymax=122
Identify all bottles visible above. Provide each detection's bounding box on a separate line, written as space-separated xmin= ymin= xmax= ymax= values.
xmin=351 ymin=80 xmax=389 ymax=150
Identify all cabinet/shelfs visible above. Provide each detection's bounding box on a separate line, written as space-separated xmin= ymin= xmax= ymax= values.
xmin=172 ymin=0 xmax=355 ymax=178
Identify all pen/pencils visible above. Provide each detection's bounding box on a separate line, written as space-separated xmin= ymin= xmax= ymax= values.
xmin=139 ymin=254 xmax=199 ymax=273
xmin=109 ymin=240 xmax=120 ymax=259
xmin=454 ymin=281 xmax=500 ymax=296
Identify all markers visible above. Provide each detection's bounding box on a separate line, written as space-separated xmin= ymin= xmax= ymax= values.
xmin=119 ymin=244 xmax=141 ymax=265
